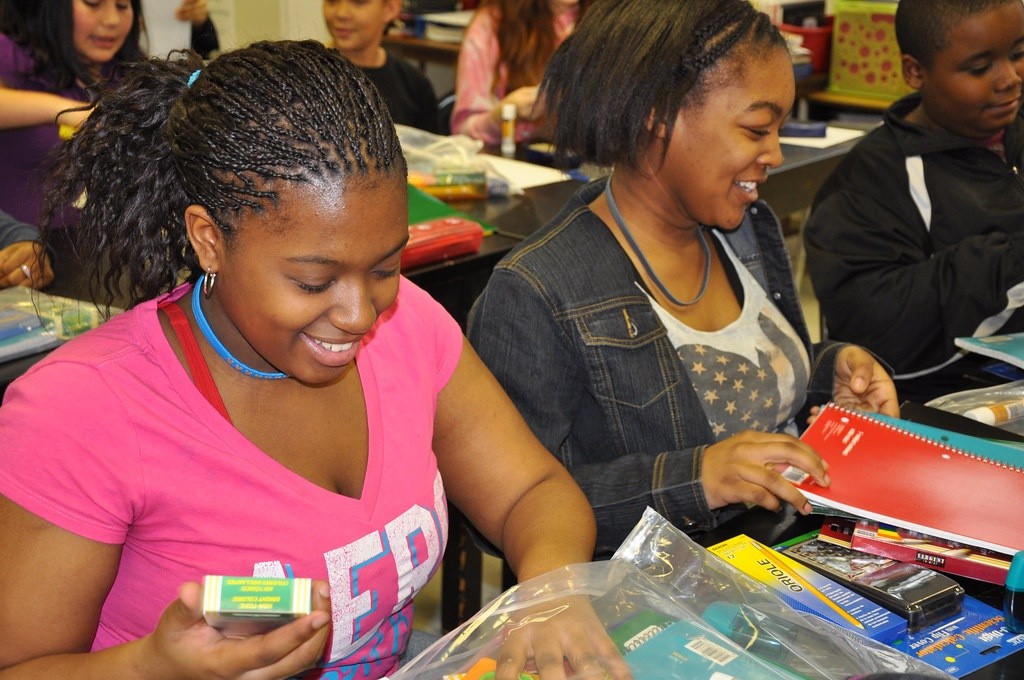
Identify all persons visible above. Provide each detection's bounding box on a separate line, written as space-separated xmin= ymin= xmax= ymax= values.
xmin=465 ymin=1 xmax=900 ymax=555
xmin=803 ymin=0 xmax=1024 ymax=404
xmin=0 ymin=39 xmax=632 ymax=680
xmin=0 ymin=0 xmax=151 ymax=229
xmin=0 ymin=211 xmax=54 ymax=292
xmin=175 ymin=0 xmax=597 ymax=143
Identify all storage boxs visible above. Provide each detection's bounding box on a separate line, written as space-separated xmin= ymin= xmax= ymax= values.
xmin=825 ymin=0 xmax=918 ymax=102
xmin=202 ymin=574 xmax=313 ymax=636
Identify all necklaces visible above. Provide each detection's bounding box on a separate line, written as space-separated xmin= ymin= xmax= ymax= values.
xmin=605 ymin=174 xmax=712 ymax=306
xmin=192 ymin=274 xmax=288 ymax=380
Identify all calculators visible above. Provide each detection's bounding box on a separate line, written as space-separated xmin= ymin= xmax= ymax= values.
xmin=779 ymin=536 xmax=965 ymax=634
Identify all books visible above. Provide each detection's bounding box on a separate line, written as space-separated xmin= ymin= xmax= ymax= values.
xmin=777 ymin=401 xmax=1024 ymax=587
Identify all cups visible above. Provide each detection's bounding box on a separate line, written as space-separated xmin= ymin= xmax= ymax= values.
xmin=1003 ymin=549 xmax=1024 ymax=634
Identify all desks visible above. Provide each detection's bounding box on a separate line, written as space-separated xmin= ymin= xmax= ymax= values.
xmin=807 ymin=91 xmax=891 ymax=123
xmin=0 ymin=229 xmax=517 ymax=633
xmin=445 ymin=121 xmax=875 ymax=593
xmin=380 ymin=37 xmax=461 ymax=71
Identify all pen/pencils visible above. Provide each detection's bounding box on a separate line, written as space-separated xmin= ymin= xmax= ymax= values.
xmin=750 ymin=540 xmax=866 ymax=630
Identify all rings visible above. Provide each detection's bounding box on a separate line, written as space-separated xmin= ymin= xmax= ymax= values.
xmin=21 ymin=265 xmax=31 ymax=279
